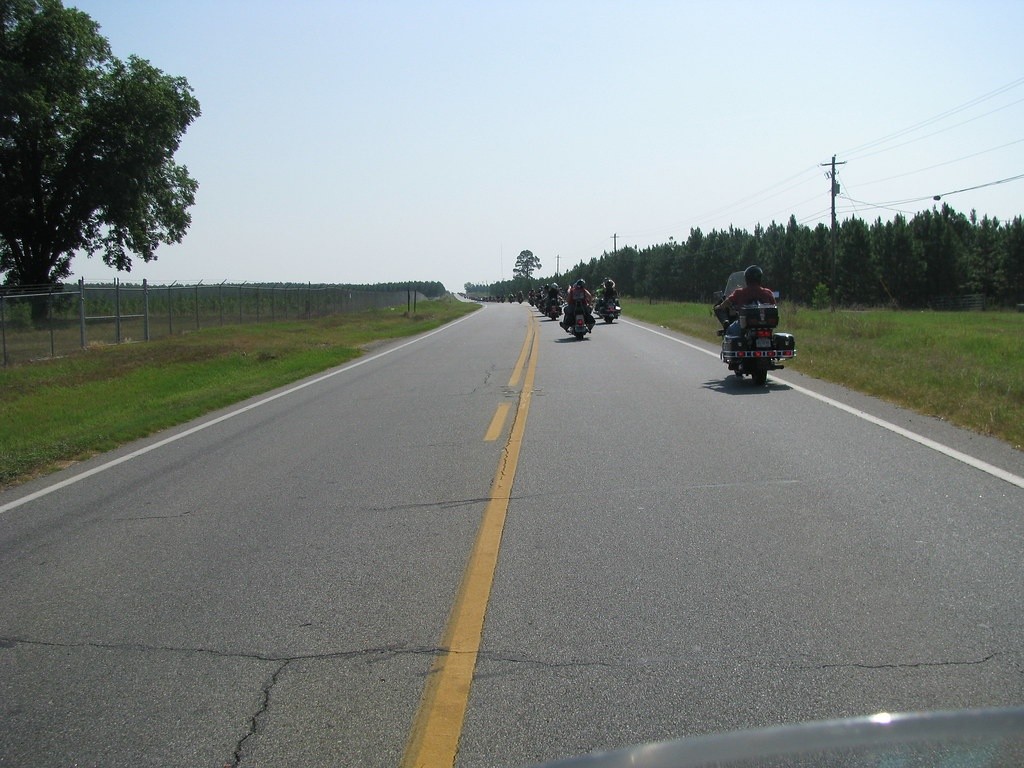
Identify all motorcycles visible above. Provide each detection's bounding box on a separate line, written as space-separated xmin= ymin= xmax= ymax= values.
xmin=473 ymin=293 xmax=567 ymax=322
xmin=713 ymin=270 xmax=798 ymax=385
xmin=565 ymin=301 xmax=590 ymax=340
xmin=592 ymin=288 xmax=623 ymax=324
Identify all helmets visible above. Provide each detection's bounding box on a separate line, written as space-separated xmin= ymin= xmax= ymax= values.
xmin=551 ymin=282 xmax=560 ymax=289
xmin=605 ymin=279 xmax=614 ymax=285
xmin=538 ymin=284 xmax=549 ymax=290
xmin=744 ymin=264 xmax=763 ymax=278
xmin=577 ymin=279 xmax=586 ymax=287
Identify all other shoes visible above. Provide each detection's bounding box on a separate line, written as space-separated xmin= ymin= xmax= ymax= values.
xmin=559 ymin=321 xmax=568 ymax=330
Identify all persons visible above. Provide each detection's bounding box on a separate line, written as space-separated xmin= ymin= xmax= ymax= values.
xmin=593 ymin=280 xmax=620 ymax=314
xmin=528 ymin=283 xmax=563 ymax=317
xmin=559 ymin=279 xmax=596 ymax=333
xmin=714 ymin=265 xmax=776 ymax=339
xmin=475 ymin=291 xmax=524 ymax=302
